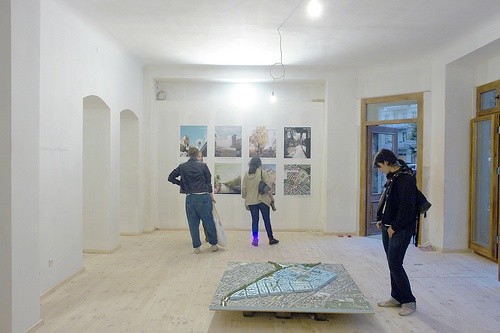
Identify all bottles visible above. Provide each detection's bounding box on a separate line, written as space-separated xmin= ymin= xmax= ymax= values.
xmin=270 ymin=201 xmax=276 ymax=211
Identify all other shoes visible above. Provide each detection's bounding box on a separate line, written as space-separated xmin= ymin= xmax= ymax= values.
xmin=269 ymin=238 xmax=279 ymax=245
xmin=378 ymin=297 xmax=401 ymax=307
xmin=195 ymin=247 xmax=200 ymax=253
xmin=399 ymin=301 xmax=416 ymax=316
xmin=211 ymin=245 xmax=218 ymax=250
xmin=252 ymin=238 xmax=259 ymax=246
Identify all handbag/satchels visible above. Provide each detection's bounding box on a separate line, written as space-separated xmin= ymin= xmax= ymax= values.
xmin=259 ymin=170 xmax=269 ymax=194
xmin=417 ymin=189 xmax=431 ymax=215
xmin=206 ymin=203 xmax=228 ymax=246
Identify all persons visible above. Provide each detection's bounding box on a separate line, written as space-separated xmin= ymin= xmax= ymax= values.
xmin=168 ymin=147 xmax=224 ymax=254
xmin=242 ymin=157 xmax=279 ymax=246
xmin=373 ymin=149 xmax=417 ymax=316
